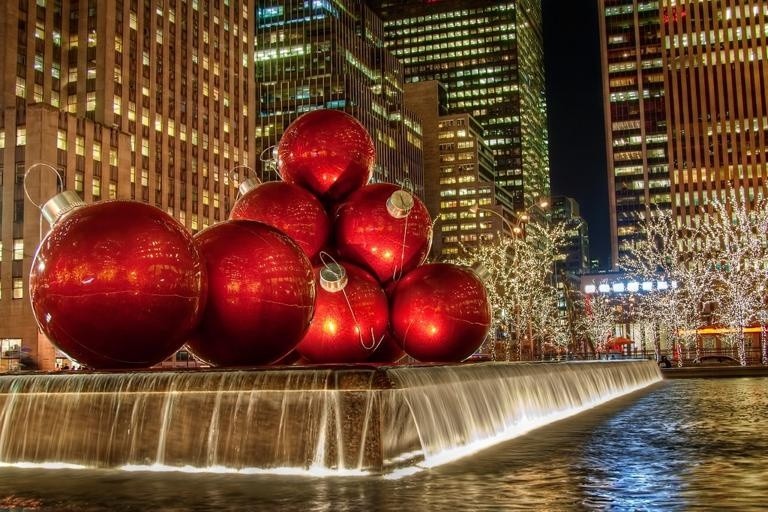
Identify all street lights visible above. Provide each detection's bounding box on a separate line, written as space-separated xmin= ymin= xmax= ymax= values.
xmin=468 ymin=196 xmax=549 ymax=361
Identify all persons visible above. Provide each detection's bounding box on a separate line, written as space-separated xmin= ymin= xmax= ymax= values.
xmin=61 ymin=362 xmax=69 ymax=369
xmin=71 ymin=366 xmax=76 ymax=371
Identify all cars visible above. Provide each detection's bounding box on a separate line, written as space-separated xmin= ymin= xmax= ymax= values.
xmin=692 ymin=355 xmax=743 ymax=366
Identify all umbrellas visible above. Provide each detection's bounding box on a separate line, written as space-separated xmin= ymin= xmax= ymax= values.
xmin=607 ymin=336 xmax=636 ymax=356
